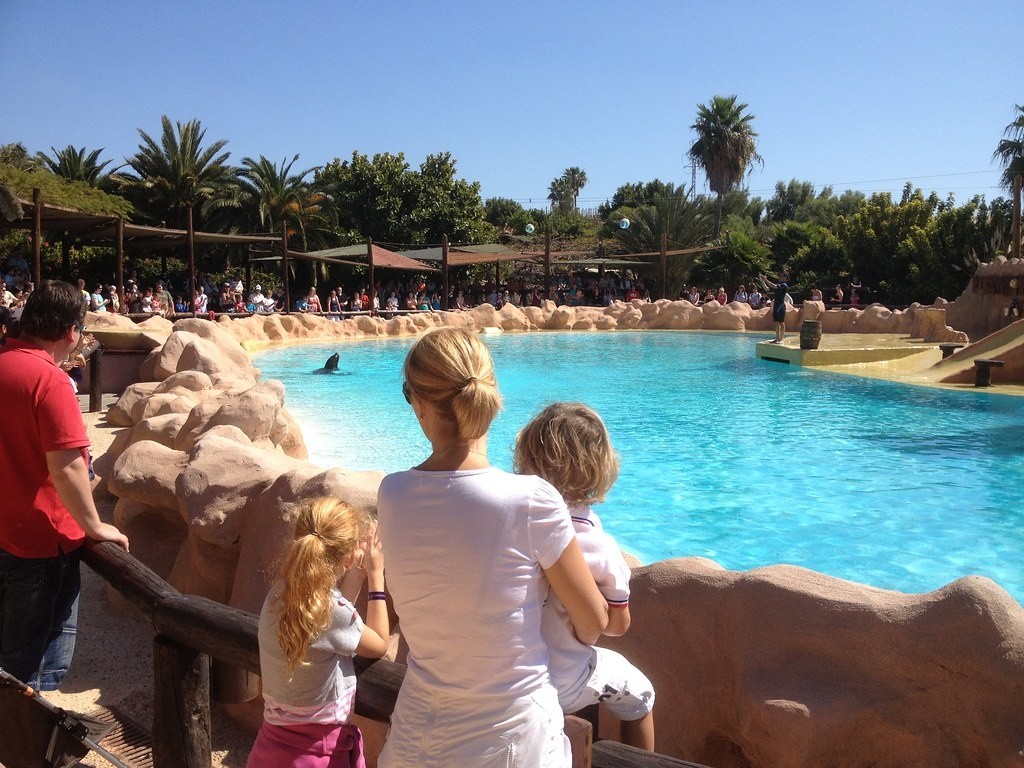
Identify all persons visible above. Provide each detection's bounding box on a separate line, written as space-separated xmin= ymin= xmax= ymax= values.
xmin=0 ymin=279 xmax=129 ymax=690
xmin=830 ymin=284 xmax=844 ymax=303
xmin=679 ymin=283 xmax=793 ymax=306
xmin=470 ymin=273 xmax=651 ymax=308
xmin=511 ymin=403 xmax=655 ymax=753
xmin=0 ymin=246 xmax=286 ymax=404
xmin=847 ymin=274 xmax=862 ymax=306
xmin=810 ymin=285 xmax=822 ymax=301
xmin=292 ymin=276 xmax=469 ymax=321
xmin=378 ymin=328 xmax=610 ymax=768
xmin=244 ymin=498 xmax=390 ymax=768
xmin=758 ymin=271 xmax=790 ymax=344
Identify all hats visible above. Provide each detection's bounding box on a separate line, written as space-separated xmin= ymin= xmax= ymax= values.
xmin=255 ymin=284 xmax=261 ymax=291
xmin=223 ymin=283 xmax=230 ymax=286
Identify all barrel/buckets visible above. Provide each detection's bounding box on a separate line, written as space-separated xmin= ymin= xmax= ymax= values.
xmin=799 ymin=319 xmax=822 ymax=349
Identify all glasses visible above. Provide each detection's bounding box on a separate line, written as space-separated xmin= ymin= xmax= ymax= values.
xmin=402 ymin=381 xmax=416 ymax=405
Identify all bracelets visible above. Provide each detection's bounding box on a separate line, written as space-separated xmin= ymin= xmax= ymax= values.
xmin=367 ymin=592 xmax=387 ymax=600
